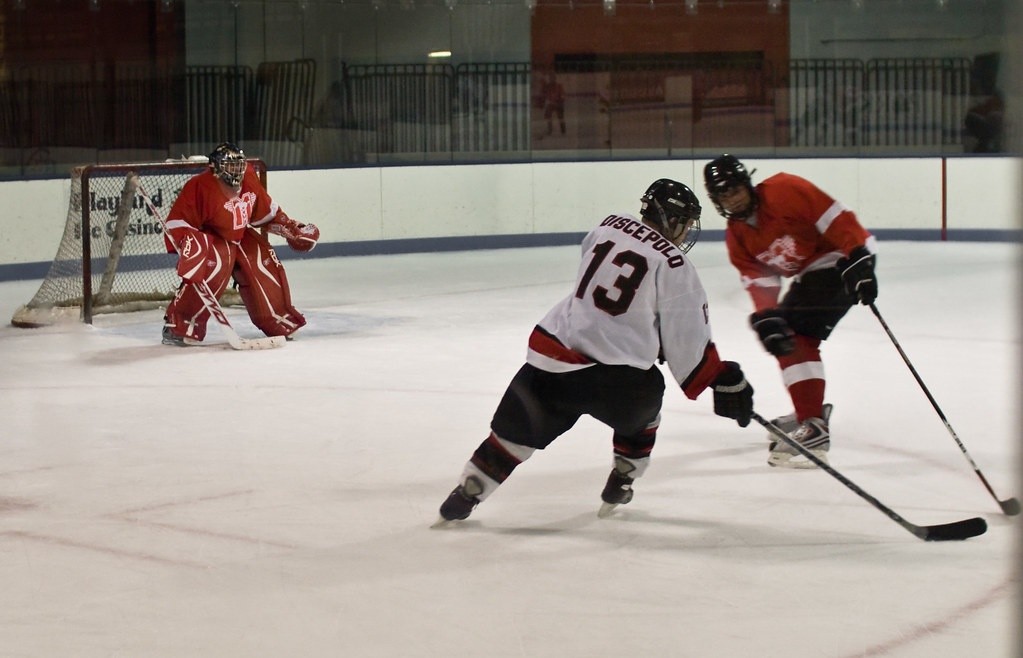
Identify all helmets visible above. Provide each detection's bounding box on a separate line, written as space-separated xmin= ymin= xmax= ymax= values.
xmin=704 ymin=153 xmax=759 ymax=219
xmin=210 ymin=142 xmax=248 ymax=186
xmin=640 ymin=178 xmax=702 ymax=253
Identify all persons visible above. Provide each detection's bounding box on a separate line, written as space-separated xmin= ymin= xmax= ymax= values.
xmin=701 ymin=151 xmax=880 ymax=470
xmin=429 ymin=176 xmax=756 ymax=532
xmin=159 ymin=140 xmax=322 ymax=350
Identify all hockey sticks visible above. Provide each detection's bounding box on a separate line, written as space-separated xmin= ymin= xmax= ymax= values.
xmin=706 ymin=381 xmax=990 ymax=543
xmin=130 ymin=174 xmax=288 ymax=352
xmin=867 ymin=299 xmax=1023 ymax=518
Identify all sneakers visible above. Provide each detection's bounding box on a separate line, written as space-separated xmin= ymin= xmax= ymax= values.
xmin=769 ymin=411 xmax=800 ymax=441
xmin=597 ymin=469 xmax=634 ymax=517
xmin=162 ymin=327 xmax=186 ymax=346
xmin=430 ymin=486 xmax=480 ymax=528
xmin=767 ymin=404 xmax=834 ymax=469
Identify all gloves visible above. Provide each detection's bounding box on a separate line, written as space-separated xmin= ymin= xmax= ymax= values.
xmin=750 ymin=307 xmax=795 ymax=355
xmin=835 ymin=245 xmax=879 ymax=306
xmin=713 ymin=360 xmax=753 ymax=427
